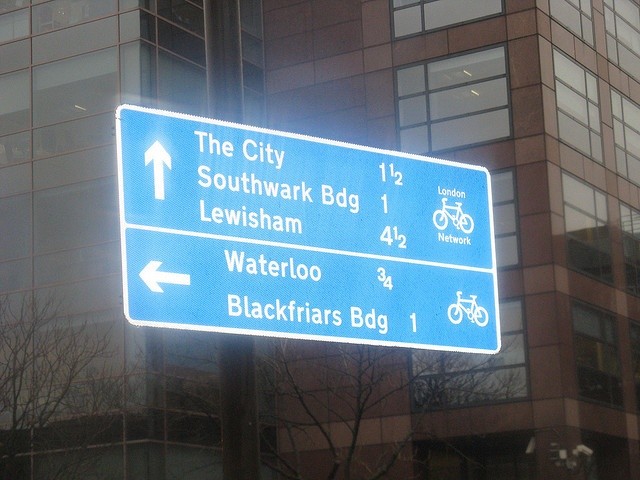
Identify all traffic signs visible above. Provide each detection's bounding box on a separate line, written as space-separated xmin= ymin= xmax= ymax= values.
xmin=121 ymin=224 xmax=501 ymax=356
xmin=116 ymin=103 xmax=497 ymax=271
